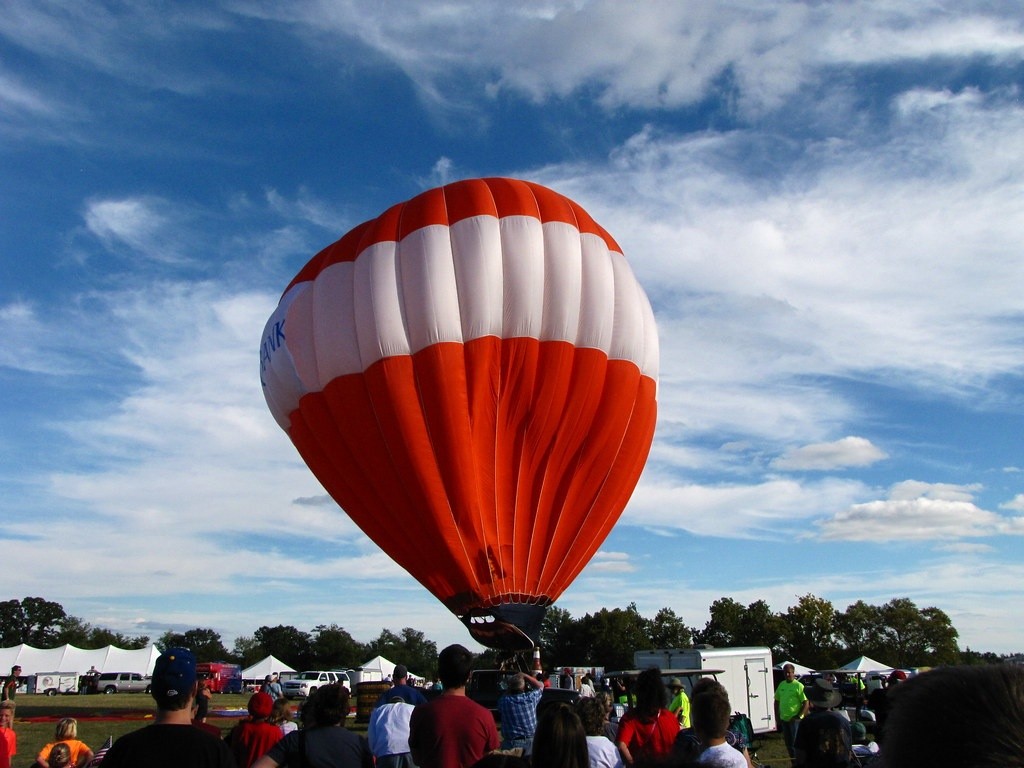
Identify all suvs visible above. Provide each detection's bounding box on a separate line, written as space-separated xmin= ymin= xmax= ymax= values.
xmin=281 ymin=670 xmax=352 ymax=700
xmin=95 ymin=671 xmax=152 ymax=695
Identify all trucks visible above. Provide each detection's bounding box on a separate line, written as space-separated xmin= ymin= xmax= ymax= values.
xmin=196 ymin=662 xmax=243 ymax=695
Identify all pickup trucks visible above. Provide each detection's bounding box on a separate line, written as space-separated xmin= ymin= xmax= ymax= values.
xmin=465 ymin=669 xmax=530 ymax=714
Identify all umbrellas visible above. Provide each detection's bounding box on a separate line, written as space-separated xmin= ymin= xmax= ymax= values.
xmin=836 ymin=656 xmax=894 ymax=673
xmin=773 ymin=660 xmax=821 ymax=680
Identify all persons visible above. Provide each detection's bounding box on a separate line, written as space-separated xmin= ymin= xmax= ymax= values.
xmin=496 ymin=671 xmax=544 ymax=756
xmin=224 ymin=692 xmax=284 ymax=768
xmin=250 ymin=683 xmax=376 ymax=768
xmin=772 ymin=663 xmax=1024 ymax=768
xmin=38 ymin=718 xmax=94 ymax=768
xmin=266 ymin=697 xmax=298 ymax=736
xmin=1 ymin=665 xmax=21 ymax=729
xmin=0 ymin=699 xmax=17 ymax=768
xmin=472 ymin=667 xmax=756 ymax=768
xmin=195 ymin=679 xmax=213 ymax=723
xmin=96 ymin=648 xmax=239 ymax=768
xmin=260 ymin=673 xmax=284 ymax=702
xmin=367 ymin=664 xmax=441 ymax=768
xmin=407 ymin=643 xmax=502 ymax=768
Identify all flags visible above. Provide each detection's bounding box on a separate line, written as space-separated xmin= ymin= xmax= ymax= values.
xmin=85 ymin=737 xmax=110 ymax=768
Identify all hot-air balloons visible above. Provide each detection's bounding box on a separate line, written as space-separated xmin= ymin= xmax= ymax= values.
xmin=258 ymin=176 xmax=662 ymax=690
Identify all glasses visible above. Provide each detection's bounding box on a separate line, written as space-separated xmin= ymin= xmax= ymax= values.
xmin=17 ymin=669 xmax=21 ymax=671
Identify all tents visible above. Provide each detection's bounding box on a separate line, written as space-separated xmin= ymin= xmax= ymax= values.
xmin=239 ymin=654 xmax=297 ymax=684
xmin=0 ymin=643 xmax=163 ymax=676
xmin=345 ymin=654 xmax=425 ymax=690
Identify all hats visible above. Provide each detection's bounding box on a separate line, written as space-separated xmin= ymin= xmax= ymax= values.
xmin=665 ymin=677 xmax=687 ymax=689
xmin=850 ymin=721 xmax=866 ymax=742
xmin=152 ymin=647 xmax=196 ymax=697
xmin=887 ymin=670 xmax=906 ymax=681
xmin=394 ymin=664 xmax=407 ymax=678
xmin=248 ymin=692 xmax=273 ymax=717
xmin=804 ymin=678 xmax=842 ymax=707
xmin=265 ymin=675 xmax=277 ymax=682
